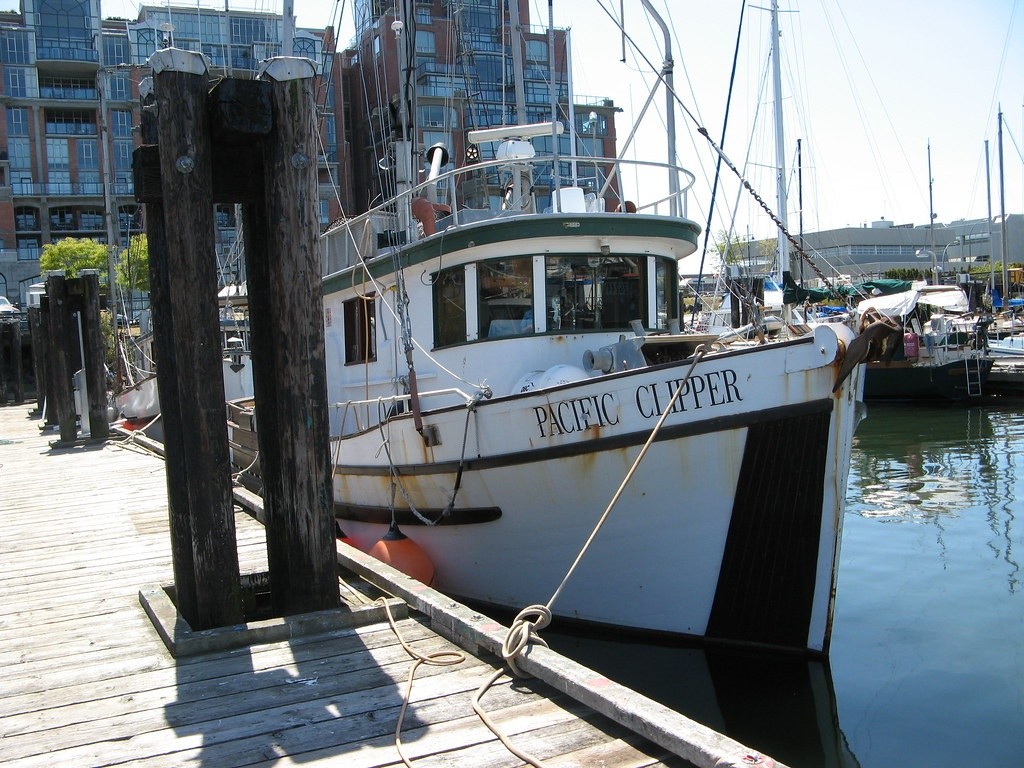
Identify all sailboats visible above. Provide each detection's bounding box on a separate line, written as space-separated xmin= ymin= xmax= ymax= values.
xmin=96 ymin=0 xmax=1024 ymax=658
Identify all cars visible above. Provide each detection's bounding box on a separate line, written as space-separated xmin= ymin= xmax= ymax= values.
xmin=0 ymin=295 xmax=22 ymax=321
